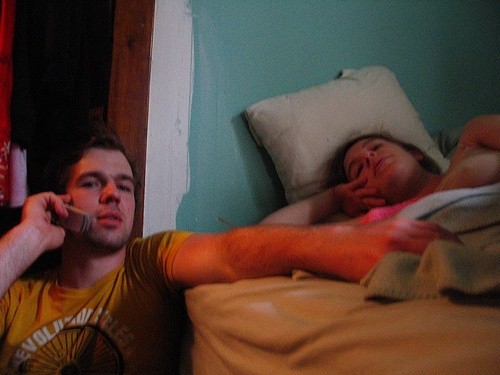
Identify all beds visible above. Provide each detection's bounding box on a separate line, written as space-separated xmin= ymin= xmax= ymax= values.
xmin=182 ymin=115 xmax=500 ymax=375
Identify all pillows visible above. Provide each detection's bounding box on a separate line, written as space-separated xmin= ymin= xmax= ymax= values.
xmin=246 ymin=65 xmax=450 ymax=281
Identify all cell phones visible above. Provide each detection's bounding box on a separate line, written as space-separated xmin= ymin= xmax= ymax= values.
xmin=52 ymin=203 xmax=91 ymax=234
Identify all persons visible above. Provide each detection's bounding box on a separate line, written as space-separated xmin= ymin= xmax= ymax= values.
xmin=0 ymin=128 xmax=465 ymax=374
xmin=254 ymin=114 xmax=500 ymax=225
